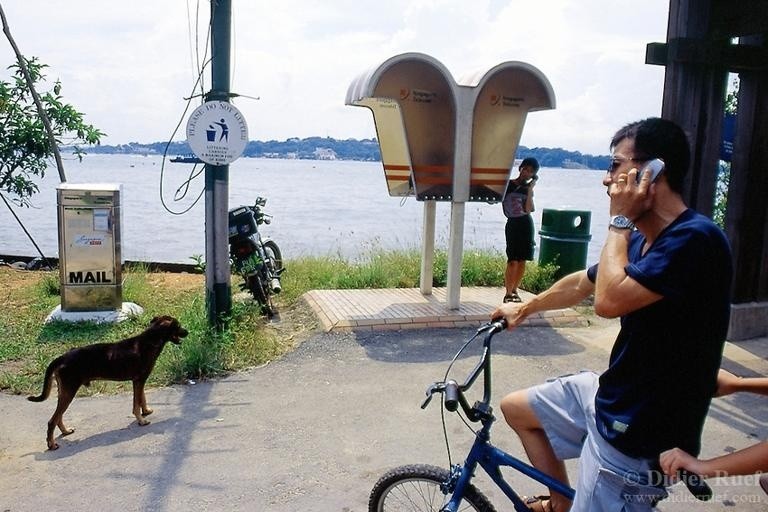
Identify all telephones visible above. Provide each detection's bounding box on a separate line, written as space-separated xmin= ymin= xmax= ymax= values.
xmin=409 ymin=176 xmax=412 ymax=188
xmin=522 ymin=174 xmax=538 ymax=194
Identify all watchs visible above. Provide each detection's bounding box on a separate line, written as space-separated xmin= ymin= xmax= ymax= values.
xmin=606 ymin=213 xmax=636 ymax=232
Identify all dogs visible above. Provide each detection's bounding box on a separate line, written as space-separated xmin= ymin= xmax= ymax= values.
xmin=26 ymin=316 xmax=189 ymax=450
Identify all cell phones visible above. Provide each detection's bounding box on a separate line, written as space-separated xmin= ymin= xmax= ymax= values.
xmin=637 ymin=157 xmax=666 ymax=185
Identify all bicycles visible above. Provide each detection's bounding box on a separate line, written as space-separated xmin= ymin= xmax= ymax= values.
xmin=366 ymin=316 xmax=715 ymax=511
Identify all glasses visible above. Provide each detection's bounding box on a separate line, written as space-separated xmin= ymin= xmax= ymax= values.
xmin=609 ymin=157 xmax=641 ymax=171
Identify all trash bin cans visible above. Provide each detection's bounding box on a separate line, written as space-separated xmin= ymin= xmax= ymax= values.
xmin=538 ymin=208 xmax=591 ymax=292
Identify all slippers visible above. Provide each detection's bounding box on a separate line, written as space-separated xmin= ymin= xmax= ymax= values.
xmin=524 ymin=496 xmax=550 ymax=512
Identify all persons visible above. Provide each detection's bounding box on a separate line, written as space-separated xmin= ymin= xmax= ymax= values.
xmin=657 ymin=363 xmax=768 ymax=510
xmin=491 ymin=118 xmax=732 ymax=511
xmin=500 ymin=157 xmax=537 ymax=303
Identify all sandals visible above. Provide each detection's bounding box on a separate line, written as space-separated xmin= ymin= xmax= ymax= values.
xmin=504 ymin=291 xmax=520 ymax=303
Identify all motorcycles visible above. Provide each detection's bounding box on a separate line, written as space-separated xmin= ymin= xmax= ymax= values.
xmin=228 ymin=194 xmax=284 ymax=318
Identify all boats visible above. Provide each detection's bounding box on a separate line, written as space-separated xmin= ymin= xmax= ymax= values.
xmin=169 ymin=153 xmax=204 ymax=164
